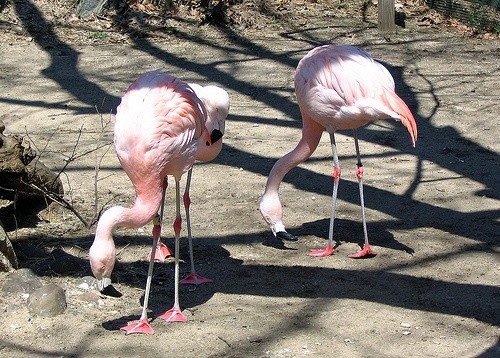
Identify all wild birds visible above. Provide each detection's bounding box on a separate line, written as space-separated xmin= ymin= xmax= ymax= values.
xmin=259 ymin=44 xmax=418 ymax=259
xmin=145 ymin=83 xmax=230 ymax=286
xmin=87 ymin=72 xmax=209 ymax=335
